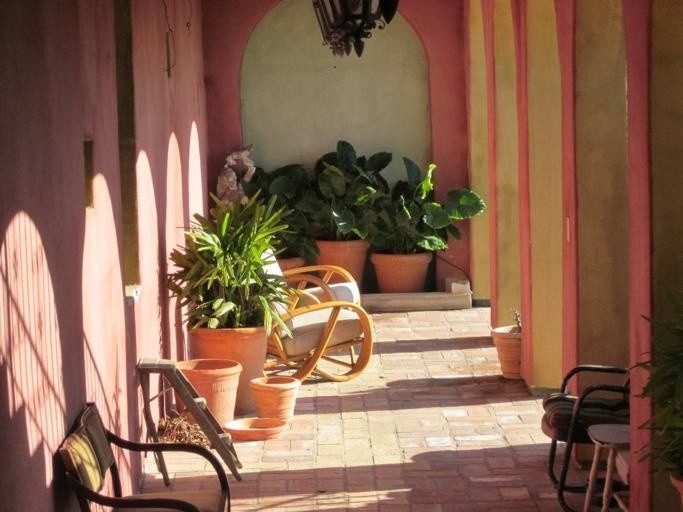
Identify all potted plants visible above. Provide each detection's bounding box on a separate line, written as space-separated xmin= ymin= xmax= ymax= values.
xmin=159 ymin=188 xmax=297 ymax=413
xmin=243 ymin=164 xmax=315 ymax=285
xmin=369 ymin=153 xmax=489 ymax=293
xmin=309 ymin=138 xmax=392 ymax=292
xmin=628 ymin=292 xmax=683 ymax=511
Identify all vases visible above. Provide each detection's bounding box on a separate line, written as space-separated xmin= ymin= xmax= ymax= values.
xmin=247 ymin=376 xmax=300 ymax=421
xmin=174 ymin=356 xmax=242 ymax=426
xmin=489 ymin=323 xmax=523 ymax=381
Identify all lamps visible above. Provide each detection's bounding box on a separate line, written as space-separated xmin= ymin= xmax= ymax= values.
xmin=308 ymin=1 xmax=398 ymax=60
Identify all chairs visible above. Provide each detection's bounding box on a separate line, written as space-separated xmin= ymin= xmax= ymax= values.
xmin=542 ymin=361 xmax=629 ymax=511
xmin=56 ymin=402 xmax=232 ymax=512
xmin=259 ymin=246 xmax=374 ymax=382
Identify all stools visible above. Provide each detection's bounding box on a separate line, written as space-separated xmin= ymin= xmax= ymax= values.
xmin=582 ymin=420 xmax=629 ymax=510
